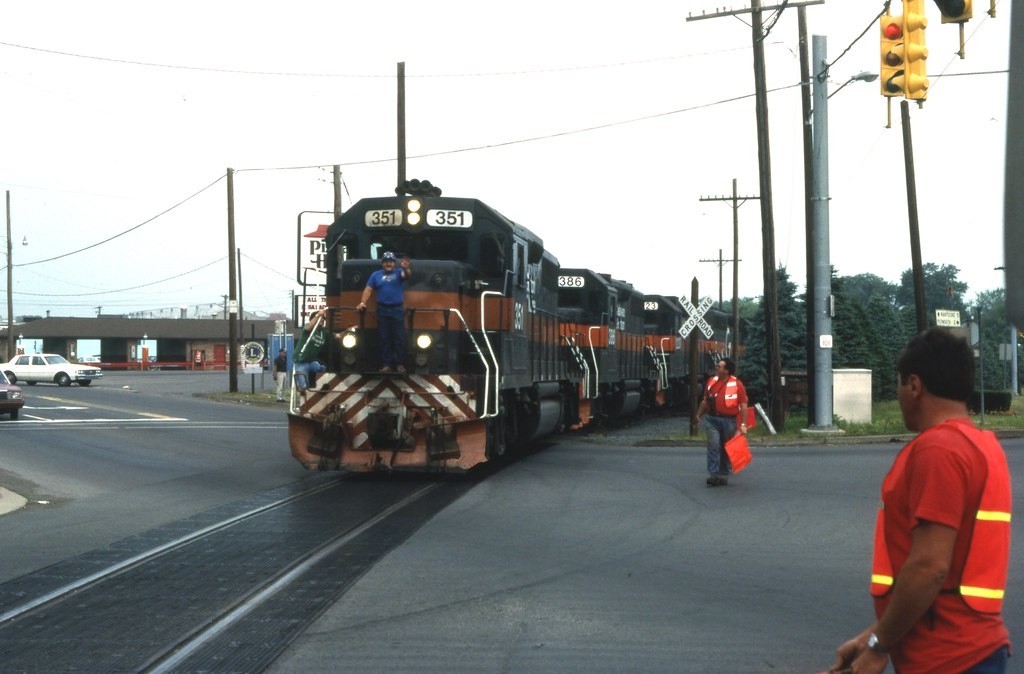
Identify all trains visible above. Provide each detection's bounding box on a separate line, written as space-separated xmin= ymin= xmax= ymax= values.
xmin=290 ymin=177 xmax=742 ymax=477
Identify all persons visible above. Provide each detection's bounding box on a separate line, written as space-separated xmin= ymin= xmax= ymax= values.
xmin=356 ymin=251 xmax=412 ymax=374
xmin=273 ymin=348 xmax=287 ymax=402
xmin=829 ymin=325 xmax=1012 ymax=674
xmin=293 ymin=309 xmax=326 ymax=389
xmin=695 ymin=357 xmax=748 ymax=486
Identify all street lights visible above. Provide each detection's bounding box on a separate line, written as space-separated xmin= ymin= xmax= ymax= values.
xmin=796 ymin=5 xmax=879 ymax=428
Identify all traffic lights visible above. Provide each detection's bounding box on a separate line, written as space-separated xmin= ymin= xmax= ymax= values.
xmin=877 ymin=16 xmax=903 ymax=99
xmin=933 ymin=0 xmax=975 ymax=25
xmin=891 ymin=1 xmax=929 ymax=105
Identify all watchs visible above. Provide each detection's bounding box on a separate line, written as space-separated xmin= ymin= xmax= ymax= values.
xmin=868 ymin=633 xmax=888 ymax=654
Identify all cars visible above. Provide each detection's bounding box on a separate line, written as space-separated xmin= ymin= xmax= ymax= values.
xmin=0 ymin=369 xmax=25 ymax=420
xmin=0 ymin=353 xmax=105 ymax=387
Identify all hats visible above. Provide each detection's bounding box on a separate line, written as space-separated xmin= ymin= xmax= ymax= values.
xmin=310 ymin=312 xmax=328 ymax=321
xmin=381 ymin=251 xmax=396 ymax=263
xmin=279 ymin=348 xmax=287 ymax=353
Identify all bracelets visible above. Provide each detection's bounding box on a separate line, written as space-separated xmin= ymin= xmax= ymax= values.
xmin=741 ymin=423 xmax=746 ymax=426
xmin=360 ymin=302 xmax=365 ymax=304
xmin=315 ymin=313 xmax=319 ymax=317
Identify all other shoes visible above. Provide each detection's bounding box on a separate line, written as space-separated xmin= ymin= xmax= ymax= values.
xmin=396 ymin=365 xmax=406 ymax=373
xmin=708 ymin=474 xmax=728 ymax=487
xmin=277 ymin=399 xmax=286 ymax=403
xmin=379 ymin=366 xmax=393 ymax=372
